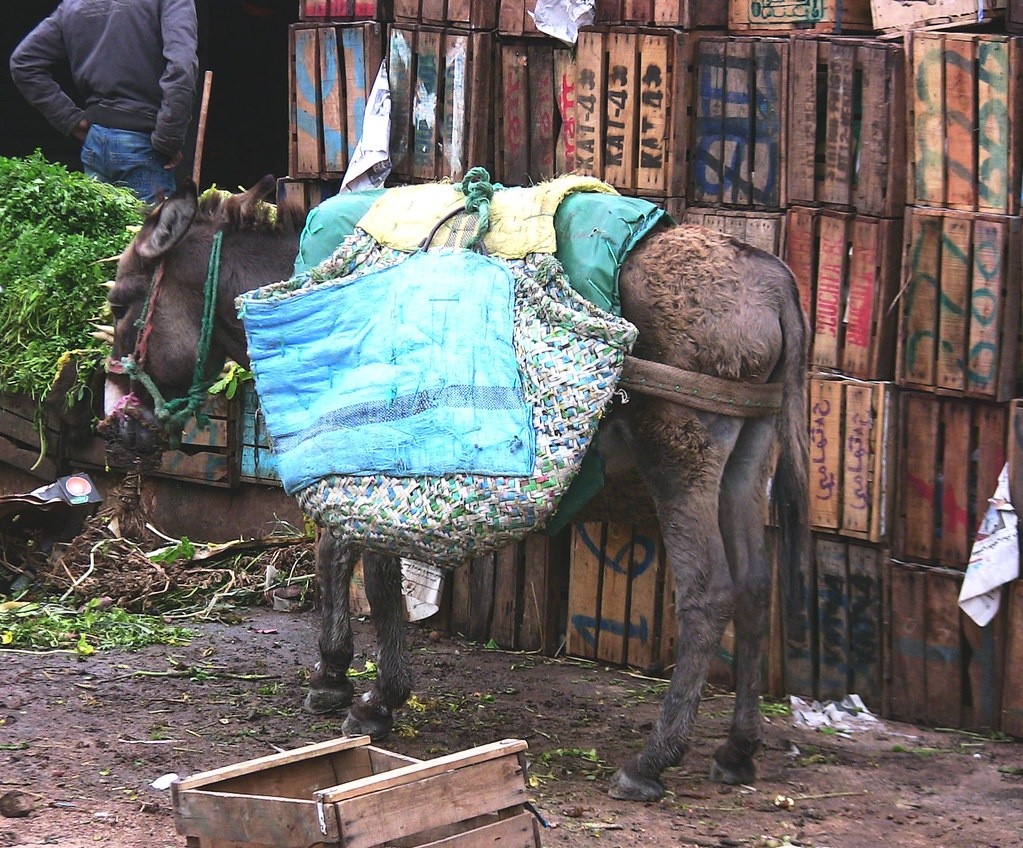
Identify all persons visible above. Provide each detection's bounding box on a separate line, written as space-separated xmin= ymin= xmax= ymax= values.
xmin=9 ymin=0 xmax=199 ymax=206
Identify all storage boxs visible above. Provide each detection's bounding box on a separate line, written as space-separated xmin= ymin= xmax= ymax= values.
xmin=170 ymin=735 xmax=548 ymax=848
xmin=0 ymin=0 xmax=1022 ymax=737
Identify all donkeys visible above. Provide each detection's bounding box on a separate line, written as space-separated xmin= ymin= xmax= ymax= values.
xmin=103 ymin=175 xmax=812 ymax=803
